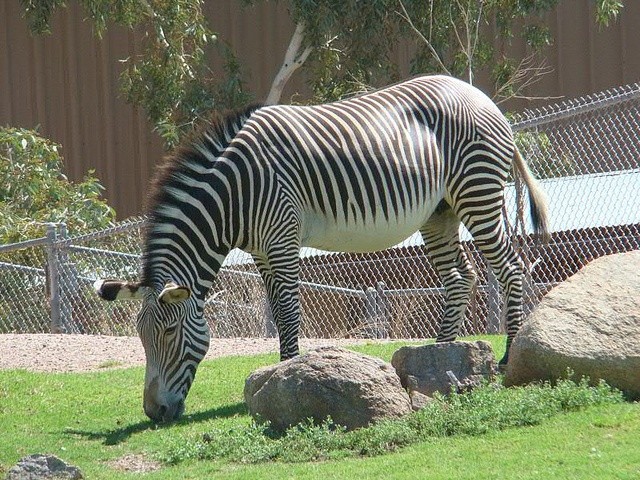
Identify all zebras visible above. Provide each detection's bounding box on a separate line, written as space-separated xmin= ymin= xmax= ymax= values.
xmin=92 ymin=72 xmax=554 ymax=425
